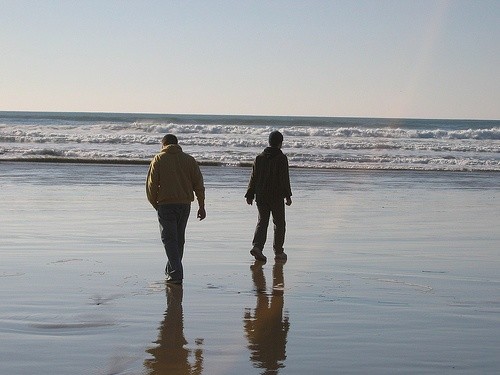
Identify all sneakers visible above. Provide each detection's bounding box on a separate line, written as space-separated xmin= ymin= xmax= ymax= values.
xmin=164 ymin=275 xmax=182 ymax=284
xmin=274 ymin=252 xmax=288 ymax=259
xmin=250 ymin=246 xmax=267 ymax=261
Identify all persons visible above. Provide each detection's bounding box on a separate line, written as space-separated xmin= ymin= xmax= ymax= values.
xmin=145 ymin=134 xmax=206 ymax=284
xmin=244 ymin=130 xmax=294 ymax=262
